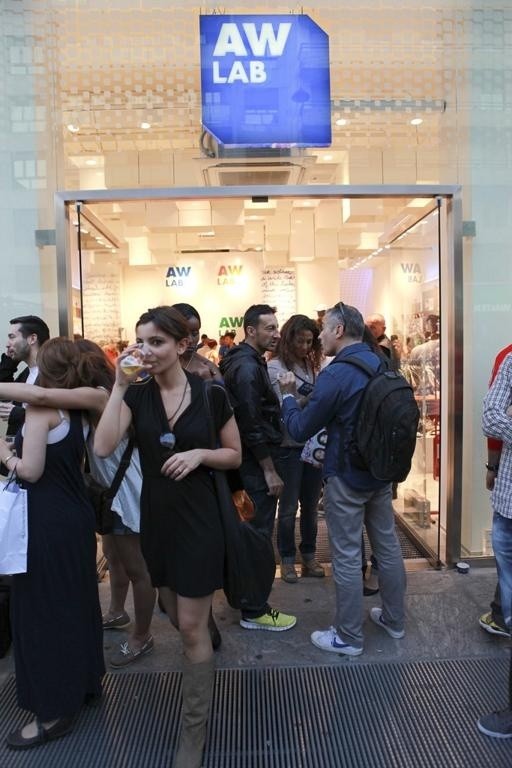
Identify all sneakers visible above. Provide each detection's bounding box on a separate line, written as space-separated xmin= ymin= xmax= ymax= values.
xmin=479 ymin=610 xmax=512 ymax=636
xmin=109 ymin=633 xmax=154 ymax=668
xmin=311 ymin=627 xmax=363 ymax=654
xmin=281 ymin=563 xmax=297 ymax=582
xmin=240 ymin=607 xmax=297 ymax=631
xmin=371 ymin=607 xmax=405 ymax=638
xmin=478 ymin=707 xmax=512 ymax=737
xmin=102 ymin=610 xmax=131 ymax=629
xmin=302 ymin=559 xmax=325 ymax=576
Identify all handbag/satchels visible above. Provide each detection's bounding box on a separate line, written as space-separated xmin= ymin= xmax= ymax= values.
xmin=81 ymin=436 xmax=136 ymax=535
xmin=0 ymin=460 xmax=27 ymax=575
xmin=202 ymin=382 xmax=278 ymax=610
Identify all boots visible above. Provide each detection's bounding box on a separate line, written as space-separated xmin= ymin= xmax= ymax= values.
xmin=171 ymin=652 xmax=214 ymax=766
xmin=208 ymin=608 xmax=221 ymax=649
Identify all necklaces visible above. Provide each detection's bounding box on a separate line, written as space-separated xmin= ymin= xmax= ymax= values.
xmin=295 ymin=358 xmax=307 ymax=373
xmin=168 ymin=379 xmax=188 ymax=423
xmin=184 ymin=354 xmax=194 ymax=370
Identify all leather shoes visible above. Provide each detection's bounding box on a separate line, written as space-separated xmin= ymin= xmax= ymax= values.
xmin=364 ymin=585 xmax=379 ymax=595
xmin=84 ymin=692 xmax=102 ymax=706
xmin=6 ymin=712 xmax=72 ymax=746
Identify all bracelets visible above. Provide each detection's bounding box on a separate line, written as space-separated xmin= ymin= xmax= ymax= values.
xmin=3 ymin=453 xmax=15 ymax=465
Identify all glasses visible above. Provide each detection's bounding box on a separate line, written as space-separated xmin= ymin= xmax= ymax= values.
xmin=335 ymin=301 xmax=347 ymax=331
xmin=159 ymin=431 xmax=175 ymax=454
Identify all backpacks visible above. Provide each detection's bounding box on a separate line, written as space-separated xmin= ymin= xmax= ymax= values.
xmin=330 ymin=342 xmax=421 ymax=483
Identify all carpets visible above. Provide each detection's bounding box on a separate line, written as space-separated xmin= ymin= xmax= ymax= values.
xmin=273 ymin=515 xmax=427 ymax=565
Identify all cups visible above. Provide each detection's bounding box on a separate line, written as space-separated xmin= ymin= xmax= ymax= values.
xmin=3 ymin=435 xmax=17 ymax=453
xmin=120 ymin=343 xmax=153 ymax=375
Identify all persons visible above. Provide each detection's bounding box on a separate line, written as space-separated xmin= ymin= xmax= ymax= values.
xmin=360 ymin=323 xmax=403 ymax=595
xmin=478 ymin=344 xmax=512 ymax=636
xmin=276 ymin=304 xmax=408 ymax=655
xmin=135 ymin=303 xmax=227 ymax=652
xmin=0 ymin=335 xmax=107 ymax=748
xmin=195 ymin=334 xmax=208 ymax=352
xmin=0 ymin=338 xmax=157 ymax=669
xmin=101 ymin=340 xmax=129 ymax=365
xmin=93 ymin=305 xmax=242 ymax=767
xmin=476 ymin=350 xmax=511 ymax=738
xmin=218 ymin=303 xmax=298 ymax=630
xmin=312 ymin=447 xmax=327 ymax=464
xmin=317 ymin=430 xmax=327 ymax=446
xmin=365 ymin=313 xmax=393 ymax=368
xmin=0 ymin=314 xmax=50 ymax=661
xmin=205 ymin=339 xmax=220 ymax=367
xmin=222 ymin=332 xmax=238 ymax=358
xmin=218 ymin=335 xmax=225 ymax=359
xmin=196 ymin=338 xmax=211 ymax=357
xmin=266 ymin=314 xmax=327 ymax=582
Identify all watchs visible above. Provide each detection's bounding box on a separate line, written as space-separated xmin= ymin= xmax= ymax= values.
xmin=485 ymin=462 xmax=500 ymax=471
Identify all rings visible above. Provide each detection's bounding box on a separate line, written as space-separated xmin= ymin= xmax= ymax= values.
xmin=177 ymin=467 xmax=182 ymax=473
